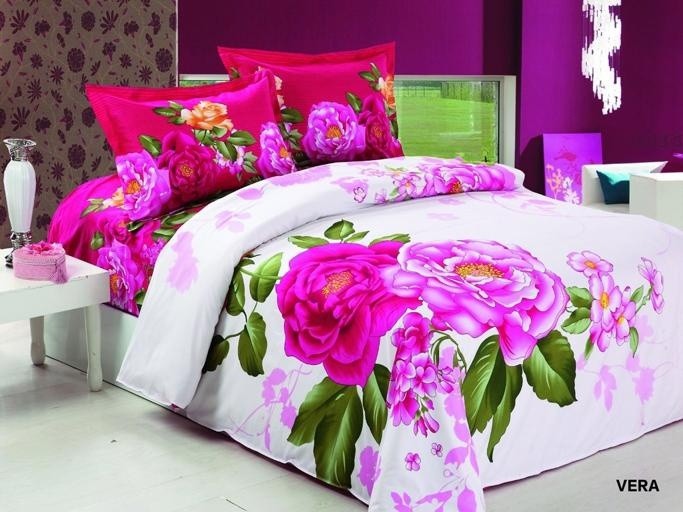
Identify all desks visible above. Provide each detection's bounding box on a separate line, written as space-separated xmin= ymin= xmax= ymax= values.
xmin=0 ymin=247 xmax=109 ymax=392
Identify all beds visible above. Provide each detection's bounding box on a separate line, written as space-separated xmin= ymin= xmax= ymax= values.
xmin=43 ymin=174 xmax=683 ymax=512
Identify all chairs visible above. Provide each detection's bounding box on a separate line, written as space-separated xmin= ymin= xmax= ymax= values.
xmin=581 ymin=160 xmax=683 ymax=215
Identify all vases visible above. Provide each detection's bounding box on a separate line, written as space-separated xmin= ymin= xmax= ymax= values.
xmin=1 ymin=137 xmax=36 ymax=267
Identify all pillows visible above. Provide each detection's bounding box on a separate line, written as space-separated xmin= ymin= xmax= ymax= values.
xmin=661 ymin=153 xmax=683 ymax=173
xmin=594 ymin=169 xmax=633 ymax=205
xmin=82 ymin=40 xmax=404 ymax=234
